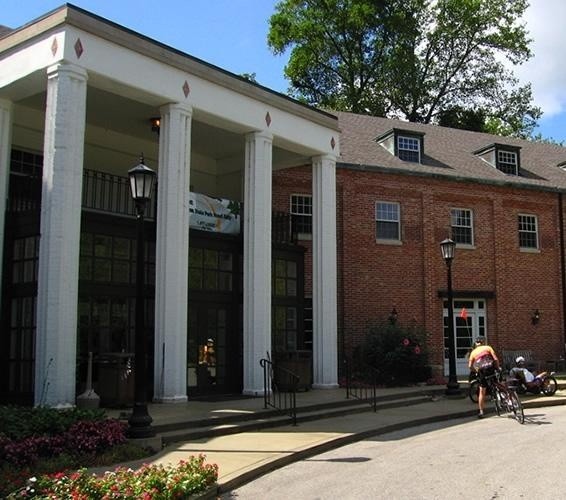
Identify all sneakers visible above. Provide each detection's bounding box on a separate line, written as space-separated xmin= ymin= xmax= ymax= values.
xmin=478 ymin=413 xmax=483 ymax=418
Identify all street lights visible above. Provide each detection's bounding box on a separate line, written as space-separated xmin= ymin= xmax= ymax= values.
xmin=437 ymin=235 xmax=461 ymax=398
xmin=127 ymin=152 xmax=156 ymax=440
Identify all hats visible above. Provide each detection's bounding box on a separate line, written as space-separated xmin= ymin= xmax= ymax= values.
xmin=515 ymin=355 xmax=526 ymax=363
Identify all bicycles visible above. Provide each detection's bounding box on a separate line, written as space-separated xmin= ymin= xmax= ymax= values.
xmin=468 ymin=367 xmax=525 ymax=425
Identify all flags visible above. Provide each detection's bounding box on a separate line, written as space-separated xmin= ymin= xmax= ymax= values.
xmin=460 ymin=307 xmax=468 ymax=320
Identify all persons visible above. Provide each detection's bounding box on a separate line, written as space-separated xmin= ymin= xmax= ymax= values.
xmin=468 ymin=337 xmax=502 ymax=418
xmin=509 ymin=356 xmax=556 ymax=388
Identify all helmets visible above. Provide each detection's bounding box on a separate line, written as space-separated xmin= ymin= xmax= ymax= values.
xmin=473 ymin=336 xmax=487 ymax=343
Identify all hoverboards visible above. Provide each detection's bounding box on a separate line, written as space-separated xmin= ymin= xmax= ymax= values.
xmin=510 ymin=365 xmax=557 ymax=396
